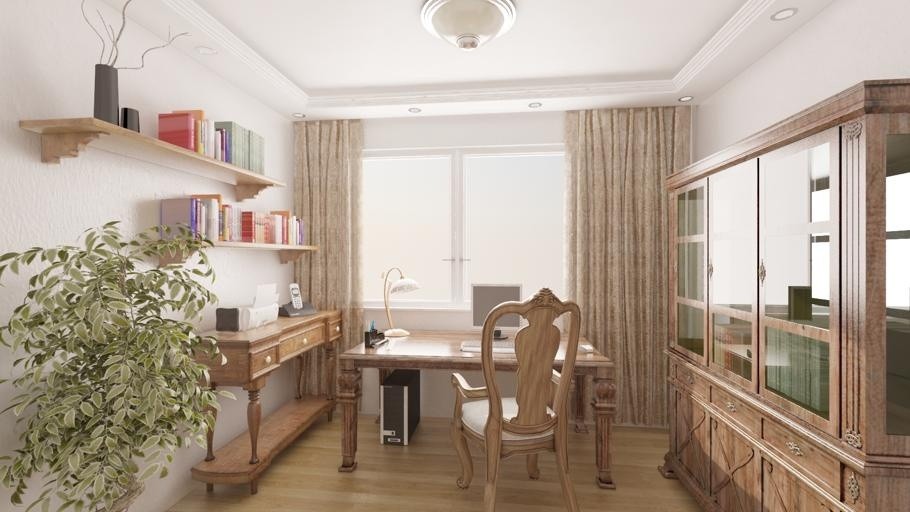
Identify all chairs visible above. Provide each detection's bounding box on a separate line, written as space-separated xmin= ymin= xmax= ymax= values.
xmin=451 ymin=288 xmax=581 ymax=512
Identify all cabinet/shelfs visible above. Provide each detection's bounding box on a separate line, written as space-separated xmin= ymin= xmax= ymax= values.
xmin=147 ymin=235 xmax=320 ymax=268
xmin=657 ymin=78 xmax=910 ymax=512
xmin=18 ymin=118 xmax=288 ymax=203
xmin=191 ymin=309 xmax=343 ymax=495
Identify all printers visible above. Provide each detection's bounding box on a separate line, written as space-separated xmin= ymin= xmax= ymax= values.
xmin=215 ymin=284 xmax=281 ymax=332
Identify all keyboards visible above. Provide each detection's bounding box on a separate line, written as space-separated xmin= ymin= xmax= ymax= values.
xmin=460 ymin=340 xmax=515 ymax=354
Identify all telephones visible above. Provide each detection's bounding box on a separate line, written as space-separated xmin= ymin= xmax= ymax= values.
xmin=278 ymin=282 xmax=314 ymax=317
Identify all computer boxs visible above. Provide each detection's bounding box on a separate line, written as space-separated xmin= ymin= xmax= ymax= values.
xmin=379 ymin=369 xmax=421 ymax=446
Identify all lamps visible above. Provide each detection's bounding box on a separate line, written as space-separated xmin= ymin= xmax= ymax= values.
xmin=421 ymin=0 xmax=516 ymax=51
xmin=383 ymin=266 xmax=420 ymax=338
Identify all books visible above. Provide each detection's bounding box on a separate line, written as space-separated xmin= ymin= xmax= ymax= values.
xmin=787 ymin=284 xmax=814 ymax=322
xmin=161 ymin=192 xmax=306 ymax=246
xmin=157 ymin=107 xmax=269 ymax=176
xmin=712 ymin=317 xmax=831 ymax=414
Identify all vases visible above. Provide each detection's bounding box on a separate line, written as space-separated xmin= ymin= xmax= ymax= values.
xmin=92 ymin=62 xmax=120 ymax=126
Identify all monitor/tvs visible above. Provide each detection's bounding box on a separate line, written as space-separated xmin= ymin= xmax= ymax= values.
xmin=470 ymin=284 xmax=522 ymax=340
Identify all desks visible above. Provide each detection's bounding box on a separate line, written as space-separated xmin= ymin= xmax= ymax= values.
xmin=337 ymin=328 xmax=618 ymax=490
